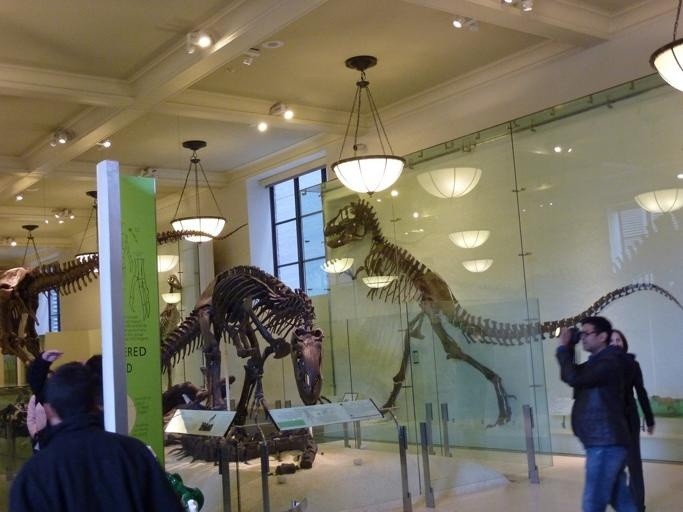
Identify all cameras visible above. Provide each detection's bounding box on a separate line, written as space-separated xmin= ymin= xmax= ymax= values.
xmin=554 ymin=327 xmax=581 ymax=344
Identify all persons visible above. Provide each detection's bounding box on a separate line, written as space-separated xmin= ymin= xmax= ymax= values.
xmin=9 ymin=350 xmax=185 ymax=512
xmin=555 ymin=316 xmax=655 ymax=512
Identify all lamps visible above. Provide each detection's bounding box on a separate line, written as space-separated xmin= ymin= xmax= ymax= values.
xmin=416 ymin=166 xmax=493 ymax=273
xmin=320 ymin=257 xmax=355 ymax=274
xmin=170 ymin=140 xmax=226 ymax=245
xmin=633 ymin=188 xmax=683 ymax=214
xmin=330 ymin=56 xmax=406 ymax=199
xmin=156 ymin=254 xmax=181 ymax=304
xmin=649 ymin=0 xmax=683 ymax=93
xmin=362 ymin=276 xmax=396 ymax=289
xmin=76 ymin=191 xmax=99 ymax=274
